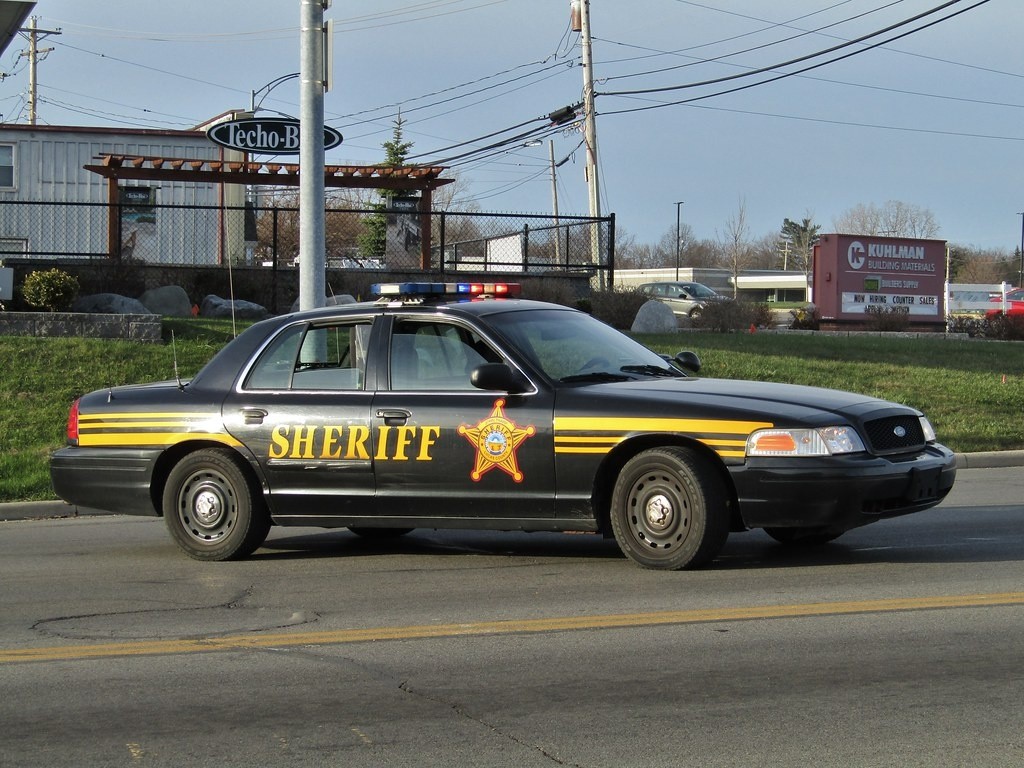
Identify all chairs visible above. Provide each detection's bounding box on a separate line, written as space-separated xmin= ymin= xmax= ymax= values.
xmin=466 ymin=339 xmax=503 ymax=374
xmin=391 ymin=343 xmax=419 ymax=386
xmin=669 ymin=288 xmax=676 ymax=298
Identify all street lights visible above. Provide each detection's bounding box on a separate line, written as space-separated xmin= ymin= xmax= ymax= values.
xmin=674 ymin=201 xmax=684 ymax=281
xmin=1017 ymin=212 xmax=1024 ymax=288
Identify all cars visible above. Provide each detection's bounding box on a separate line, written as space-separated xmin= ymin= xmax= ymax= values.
xmin=985 ymin=287 xmax=1024 ymax=319
xmin=49 ymin=283 xmax=956 ymax=571
xmin=635 ymin=282 xmax=734 ymax=319
